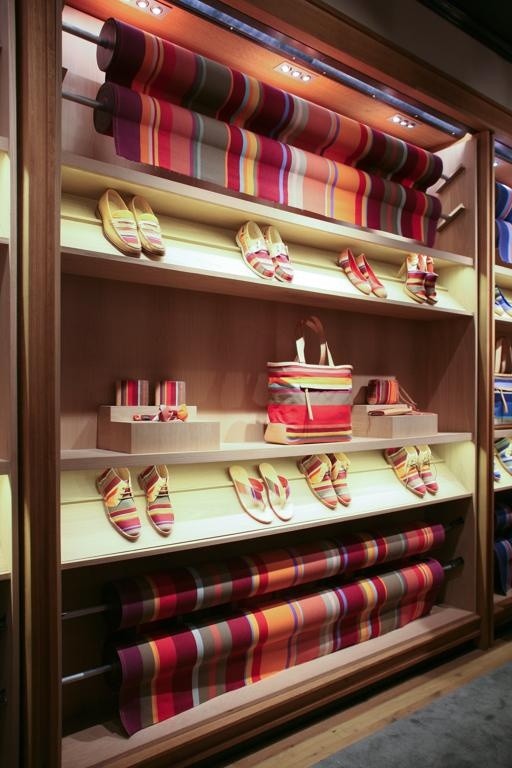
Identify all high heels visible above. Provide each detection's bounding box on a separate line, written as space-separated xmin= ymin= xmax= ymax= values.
xmin=396 ymin=252 xmax=440 ymax=305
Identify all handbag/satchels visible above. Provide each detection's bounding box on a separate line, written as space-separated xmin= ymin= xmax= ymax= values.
xmin=368 ymin=379 xmax=400 ymax=405
xmin=264 ymin=316 xmax=354 ymax=446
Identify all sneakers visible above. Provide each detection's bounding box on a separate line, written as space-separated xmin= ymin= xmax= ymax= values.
xmin=355 ymin=253 xmax=387 ymax=298
xmin=261 ymin=225 xmax=294 ymax=282
xmin=95 ymin=189 xmax=141 ymax=257
xmin=236 ymin=220 xmax=275 ymax=280
xmin=329 ymin=453 xmax=352 ymax=506
xmin=494 ymin=436 xmax=512 ymax=480
xmin=335 ymin=248 xmax=371 ymax=294
xmin=128 ymin=195 xmax=165 ymax=258
xmin=96 ymin=466 xmax=141 ymax=539
xmin=493 ymin=286 xmax=512 ymax=318
xmin=383 ymin=445 xmax=438 ymax=497
xmin=298 ymin=454 xmax=338 ymax=508
xmin=138 ymin=465 xmax=174 ymax=536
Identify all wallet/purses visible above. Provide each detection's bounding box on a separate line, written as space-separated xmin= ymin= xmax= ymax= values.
xmin=370 ymin=406 xmax=412 ymax=416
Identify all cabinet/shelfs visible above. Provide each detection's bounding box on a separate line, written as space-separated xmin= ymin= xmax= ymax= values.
xmin=1 ymin=0 xmax=511 ymax=768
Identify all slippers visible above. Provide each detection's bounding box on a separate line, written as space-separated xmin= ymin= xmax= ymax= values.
xmin=259 ymin=462 xmax=293 ymax=520
xmin=228 ymin=466 xmax=274 ymax=524
